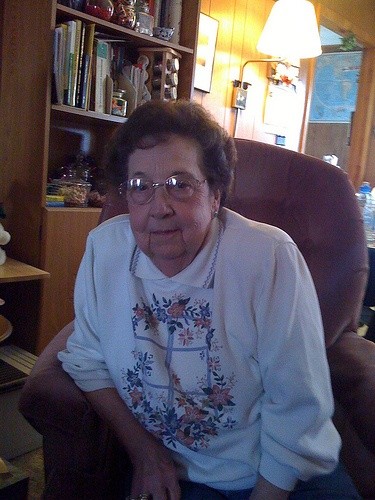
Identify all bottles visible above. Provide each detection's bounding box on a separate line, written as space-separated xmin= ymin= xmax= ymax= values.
xmin=111 ymin=89 xmax=127 ymax=117
xmin=355 ymin=181 xmax=375 ymax=249
xmin=134 ymin=7 xmax=153 ymax=37
xmin=58 ymin=159 xmax=93 ymax=208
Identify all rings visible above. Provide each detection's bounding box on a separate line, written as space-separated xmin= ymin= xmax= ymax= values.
xmin=139 ymin=492 xmax=152 ymax=500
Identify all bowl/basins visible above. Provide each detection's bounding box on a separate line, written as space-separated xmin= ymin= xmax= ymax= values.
xmin=153 ymin=27 xmax=175 ymax=42
xmin=83 ymin=0 xmax=114 ymax=21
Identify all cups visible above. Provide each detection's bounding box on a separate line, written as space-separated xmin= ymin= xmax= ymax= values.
xmin=110 ymin=0 xmax=137 ymax=30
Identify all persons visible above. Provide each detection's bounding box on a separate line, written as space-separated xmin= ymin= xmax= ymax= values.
xmin=56 ymin=97 xmax=365 ymax=500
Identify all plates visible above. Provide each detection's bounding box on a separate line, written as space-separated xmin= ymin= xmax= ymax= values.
xmin=0 ymin=314 xmax=13 ymax=341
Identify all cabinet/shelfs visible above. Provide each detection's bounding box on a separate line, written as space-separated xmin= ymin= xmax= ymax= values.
xmin=0 ymin=0 xmax=202 ymax=358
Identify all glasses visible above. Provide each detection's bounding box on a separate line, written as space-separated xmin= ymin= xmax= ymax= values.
xmin=118 ymin=176 xmax=210 ymax=205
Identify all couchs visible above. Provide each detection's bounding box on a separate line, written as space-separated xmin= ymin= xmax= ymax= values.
xmin=17 ymin=137 xmax=375 ymax=500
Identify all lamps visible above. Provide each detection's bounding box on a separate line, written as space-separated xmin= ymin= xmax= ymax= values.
xmin=230 ymin=1 xmax=324 ymax=110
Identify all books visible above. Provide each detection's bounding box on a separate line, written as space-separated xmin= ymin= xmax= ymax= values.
xmin=52 ymin=18 xmax=125 ymax=115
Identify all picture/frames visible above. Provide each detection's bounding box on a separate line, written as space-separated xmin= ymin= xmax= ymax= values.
xmin=194 ymin=11 xmax=220 ymax=93
